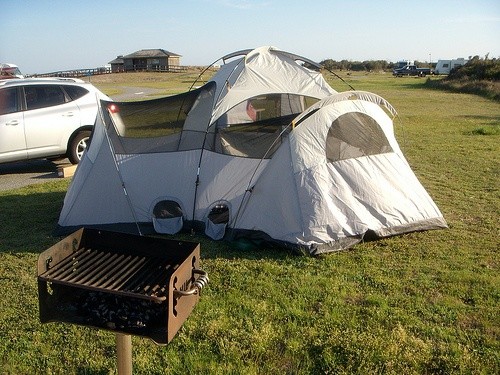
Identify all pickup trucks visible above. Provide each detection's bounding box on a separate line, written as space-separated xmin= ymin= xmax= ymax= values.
xmin=393 ymin=66 xmax=431 ymax=78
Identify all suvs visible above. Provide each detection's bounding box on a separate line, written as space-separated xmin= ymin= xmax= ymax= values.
xmin=0 ymin=78 xmax=126 ymax=166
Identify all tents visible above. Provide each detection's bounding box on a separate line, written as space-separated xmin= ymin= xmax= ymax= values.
xmin=52 ymin=46 xmax=448 ymax=256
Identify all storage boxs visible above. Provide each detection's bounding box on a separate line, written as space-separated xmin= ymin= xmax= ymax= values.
xmin=56 ymin=163 xmax=78 ymax=178
